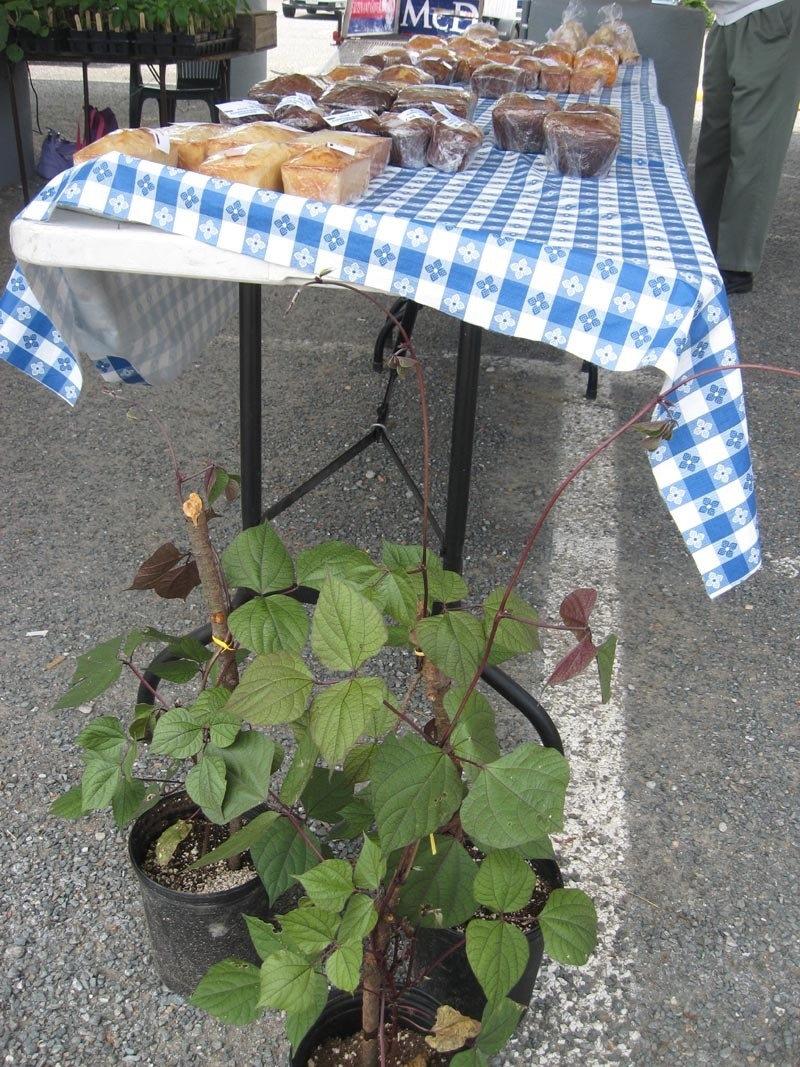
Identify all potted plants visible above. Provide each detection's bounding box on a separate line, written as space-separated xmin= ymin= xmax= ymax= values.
xmin=52 ymin=264 xmax=800 ymax=1067
xmin=0 ymin=0 xmax=251 ymax=65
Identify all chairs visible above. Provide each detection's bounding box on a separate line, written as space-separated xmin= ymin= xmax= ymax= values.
xmin=130 ymin=57 xmax=232 ymax=130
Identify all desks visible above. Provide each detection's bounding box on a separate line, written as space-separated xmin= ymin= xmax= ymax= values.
xmin=0 ymin=57 xmax=764 ymax=756
xmin=24 ymin=50 xmax=254 ymax=145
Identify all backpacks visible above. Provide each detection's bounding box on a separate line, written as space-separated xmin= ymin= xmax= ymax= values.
xmin=77 ymin=106 xmax=119 ymax=150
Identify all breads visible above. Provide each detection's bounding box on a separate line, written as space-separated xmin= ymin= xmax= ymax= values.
xmin=72 ymin=22 xmax=642 ymax=208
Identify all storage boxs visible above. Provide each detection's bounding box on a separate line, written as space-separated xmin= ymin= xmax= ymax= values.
xmin=234 ymin=12 xmax=278 ymax=50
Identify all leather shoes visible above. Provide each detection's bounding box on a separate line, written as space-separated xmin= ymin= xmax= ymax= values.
xmin=719 ymin=269 xmax=754 ymax=295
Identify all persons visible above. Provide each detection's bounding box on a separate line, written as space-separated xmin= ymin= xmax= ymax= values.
xmin=695 ymin=0 xmax=800 ymax=295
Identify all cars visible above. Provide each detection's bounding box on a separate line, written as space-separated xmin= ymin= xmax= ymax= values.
xmin=482 ymin=0 xmax=522 ymax=41
xmin=282 ymin=0 xmax=346 ymax=20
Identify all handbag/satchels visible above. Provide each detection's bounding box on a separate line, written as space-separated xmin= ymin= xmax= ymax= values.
xmin=37 ymin=127 xmax=82 ymax=180
